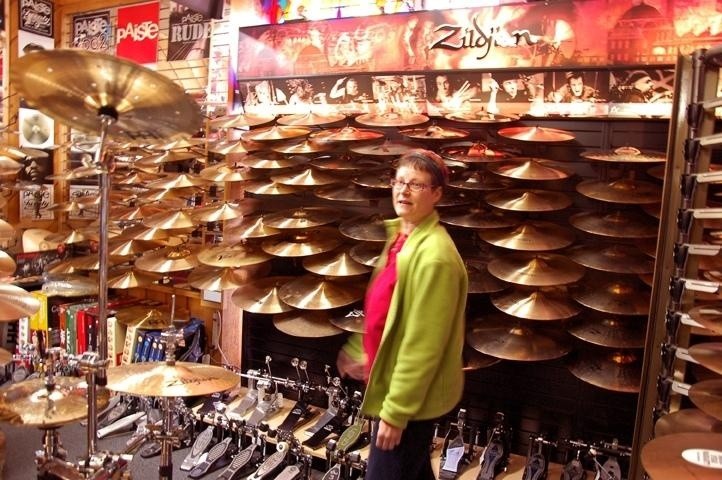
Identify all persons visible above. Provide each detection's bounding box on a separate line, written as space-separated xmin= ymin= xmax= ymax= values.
xmin=547 ymin=70 xmax=654 ymax=104
xmin=378 ymin=73 xmax=534 ymax=105
xmin=20 ymin=156 xmax=50 ymax=185
xmin=337 ymin=148 xmax=470 ymax=480
xmin=32 ymin=186 xmax=45 ymax=219
xmin=246 ymin=76 xmax=369 ymax=107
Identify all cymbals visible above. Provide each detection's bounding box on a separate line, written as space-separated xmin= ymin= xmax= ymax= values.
xmin=1 ymin=375 xmax=110 ymax=426
xmin=38 ymin=113 xmax=404 ymax=340
xmin=498 ymin=126 xmax=576 ymax=142
xmin=0 ymin=282 xmax=41 ymax=322
xmin=446 ymin=112 xmax=522 ymax=123
xmin=1 ymin=249 xmax=16 ymax=277
xmin=102 ymin=357 xmax=239 ymax=395
xmin=8 ymin=50 xmax=203 ymax=143
xmin=565 ymin=342 xmax=646 ymax=393
xmin=0 ymin=218 xmax=17 ymax=252
xmin=1 ymin=346 xmax=13 ymax=366
xmin=0 ymin=143 xmax=49 ymax=182
xmin=396 ymin=124 xmax=470 ymax=141
xmin=431 ymin=141 xmax=667 ymax=375
xmin=641 ymin=304 xmax=722 ymax=480
xmin=356 ymin=112 xmax=430 ymax=127
xmin=349 ymin=138 xmax=428 ymax=157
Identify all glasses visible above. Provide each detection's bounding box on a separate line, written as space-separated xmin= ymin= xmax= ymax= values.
xmin=389 ymin=178 xmax=438 ymax=195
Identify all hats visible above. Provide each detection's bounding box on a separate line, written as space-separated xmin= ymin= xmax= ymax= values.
xmin=624 ymin=70 xmax=649 ymax=83
